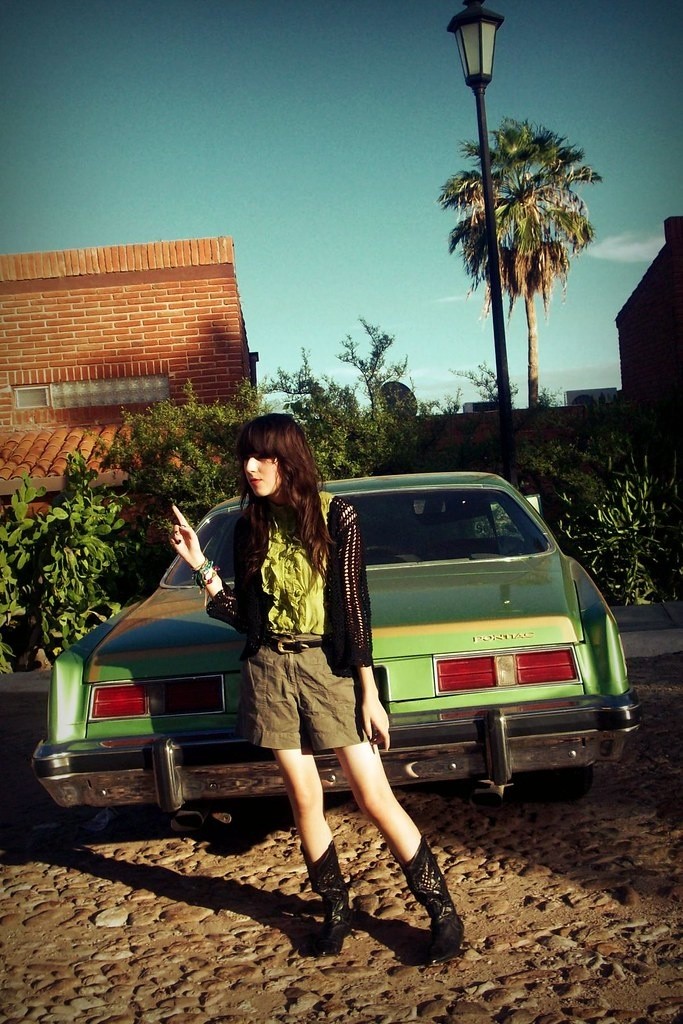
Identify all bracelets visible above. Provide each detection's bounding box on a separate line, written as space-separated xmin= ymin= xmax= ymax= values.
xmin=192 ymin=557 xmax=220 ymax=593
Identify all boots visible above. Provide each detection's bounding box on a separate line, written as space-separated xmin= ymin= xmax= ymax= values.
xmin=300 ymin=840 xmax=352 ymax=957
xmin=389 ymin=835 xmax=465 ymax=965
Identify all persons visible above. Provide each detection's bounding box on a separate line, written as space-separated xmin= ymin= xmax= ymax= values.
xmin=169 ymin=417 xmax=466 ymax=962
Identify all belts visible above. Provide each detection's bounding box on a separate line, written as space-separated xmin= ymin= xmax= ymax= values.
xmin=268 ymin=637 xmax=324 ymax=654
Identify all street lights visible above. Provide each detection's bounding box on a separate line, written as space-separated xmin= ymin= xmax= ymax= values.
xmin=443 ymin=0 xmax=520 ymax=489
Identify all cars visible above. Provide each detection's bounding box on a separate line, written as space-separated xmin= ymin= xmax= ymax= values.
xmin=28 ymin=469 xmax=645 ymax=814
